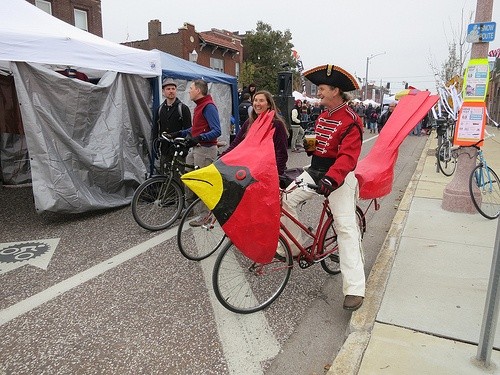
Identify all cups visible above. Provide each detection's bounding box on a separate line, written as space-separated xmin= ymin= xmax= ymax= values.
xmin=304 ymin=134 xmax=316 ymax=151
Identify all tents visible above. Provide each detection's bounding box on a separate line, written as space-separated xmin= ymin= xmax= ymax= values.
xmin=0 ymin=0 xmax=239 ymax=213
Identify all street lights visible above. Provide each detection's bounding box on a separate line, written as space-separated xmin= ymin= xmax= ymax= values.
xmin=365 ymin=51 xmax=386 ymax=100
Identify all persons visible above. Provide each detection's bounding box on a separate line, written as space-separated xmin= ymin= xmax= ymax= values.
xmin=217 ymin=90 xmax=290 ymax=175
xmin=302 ymin=100 xmax=322 ymax=120
xmin=276 ymin=64 xmax=365 ymax=310
xmin=381 ymin=102 xmax=396 ymax=128
xmin=153 ymin=78 xmax=192 ymax=207
xmin=349 ymin=102 xmax=378 ymax=133
xmin=239 ymin=93 xmax=253 ymax=127
xmin=290 ymin=99 xmax=304 ymax=151
xmin=167 ymin=80 xmax=222 ymax=227
xmin=240 ymin=84 xmax=256 ymax=101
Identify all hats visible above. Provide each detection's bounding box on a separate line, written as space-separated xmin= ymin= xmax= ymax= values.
xmin=302 ymin=64 xmax=359 ymax=92
xmin=162 ymin=78 xmax=176 ymax=89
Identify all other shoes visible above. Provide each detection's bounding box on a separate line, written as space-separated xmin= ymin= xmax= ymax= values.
xmin=158 ymin=200 xmax=176 ymax=207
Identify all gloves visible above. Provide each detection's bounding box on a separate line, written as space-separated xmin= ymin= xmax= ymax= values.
xmin=303 ymin=139 xmax=318 ymax=153
xmin=163 ymin=131 xmax=180 ymax=139
xmin=181 ymin=136 xmax=199 ymax=148
xmin=316 ymin=176 xmax=338 ymax=198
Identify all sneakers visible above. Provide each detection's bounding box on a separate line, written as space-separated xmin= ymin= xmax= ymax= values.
xmin=177 ymin=209 xmax=194 ymax=218
xmin=274 ymin=252 xmax=297 ymax=262
xmin=343 ymin=295 xmax=362 ymax=310
xmin=189 ymin=216 xmax=212 ymax=226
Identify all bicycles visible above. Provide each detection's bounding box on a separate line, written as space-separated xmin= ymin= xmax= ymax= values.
xmin=177 ymin=164 xmax=299 ymax=262
xmin=431 ymin=121 xmax=459 ymax=177
xmin=131 ymin=130 xmax=228 ymax=232
xmin=212 ymin=177 xmax=367 ymax=314
xmin=452 ymin=133 xmax=500 ymax=220
